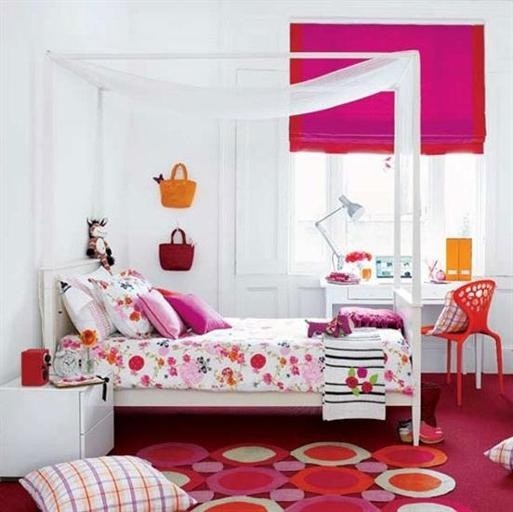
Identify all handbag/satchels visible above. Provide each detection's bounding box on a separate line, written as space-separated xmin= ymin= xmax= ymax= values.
xmin=161 ymin=177 xmax=196 ymax=208
xmin=160 ymin=241 xmax=195 ymax=271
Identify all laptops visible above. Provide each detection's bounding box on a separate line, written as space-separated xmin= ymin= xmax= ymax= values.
xmin=376 ymin=255 xmax=414 ymax=284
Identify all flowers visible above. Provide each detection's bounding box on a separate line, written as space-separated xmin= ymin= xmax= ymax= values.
xmin=78 ymin=329 xmax=97 ymax=373
xmin=344 ymin=251 xmax=371 ymax=264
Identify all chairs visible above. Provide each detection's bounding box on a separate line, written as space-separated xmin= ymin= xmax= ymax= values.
xmin=420 ymin=278 xmax=504 ymax=407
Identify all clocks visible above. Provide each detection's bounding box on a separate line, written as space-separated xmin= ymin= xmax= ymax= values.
xmin=51 ymin=348 xmax=80 ymax=377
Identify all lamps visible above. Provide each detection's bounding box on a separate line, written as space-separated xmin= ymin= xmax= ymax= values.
xmin=312 ymin=193 xmax=364 ymax=274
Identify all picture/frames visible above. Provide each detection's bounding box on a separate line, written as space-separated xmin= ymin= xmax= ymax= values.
xmin=375 ymin=255 xmax=413 ymax=279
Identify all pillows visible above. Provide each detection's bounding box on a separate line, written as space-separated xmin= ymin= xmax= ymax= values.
xmin=480 ymin=430 xmax=511 ymax=474
xmin=163 ymin=291 xmax=232 ymax=335
xmin=16 ymin=454 xmax=199 ymax=512
xmin=55 ymin=263 xmax=118 ymax=342
xmin=337 ymin=307 xmax=404 ymax=332
xmin=424 ymin=289 xmax=468 ymax=337
xmin=134 ymin=286 xmax=188 ymax=340
xmin=86 ymin=265 xmax=154 ymax=339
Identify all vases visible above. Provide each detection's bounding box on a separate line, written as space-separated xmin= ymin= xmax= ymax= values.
xmin=355 ymin=264 xmax=364 ymax=282
xmin=80 ymin=347 xmax=96 ymax=379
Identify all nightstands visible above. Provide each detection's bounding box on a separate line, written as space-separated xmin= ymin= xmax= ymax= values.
xmin=0 ymin=370 xmax=116 ymax=481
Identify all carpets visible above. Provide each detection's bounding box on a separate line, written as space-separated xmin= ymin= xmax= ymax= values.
xmin=126 ymin=442 xmax=467 ymax=512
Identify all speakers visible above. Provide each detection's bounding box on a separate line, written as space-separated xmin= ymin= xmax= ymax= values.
xmin=22 ymin=348 xmax=50 ymax=386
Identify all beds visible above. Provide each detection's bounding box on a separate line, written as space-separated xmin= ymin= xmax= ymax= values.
xmin=40 ymin=46 xmax=423 ymax=447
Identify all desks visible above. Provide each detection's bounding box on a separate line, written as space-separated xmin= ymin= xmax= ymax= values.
xmin=318 ymin=276 xmax=484 ymax=391
